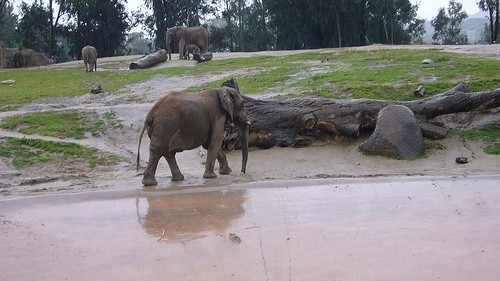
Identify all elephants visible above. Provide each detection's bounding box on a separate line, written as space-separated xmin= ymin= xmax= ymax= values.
xmin=166 ymin=26 xmax=210 ymax=60
xmin=135 ymin=86 xmax=251 ymax=186
xmin=13 ymin=50 xmax=33 ymax=68
xmin=82 ymin=45 xmax=98 ymax=72
xmin=183 ymin=44 xmax=201 ymax=60
